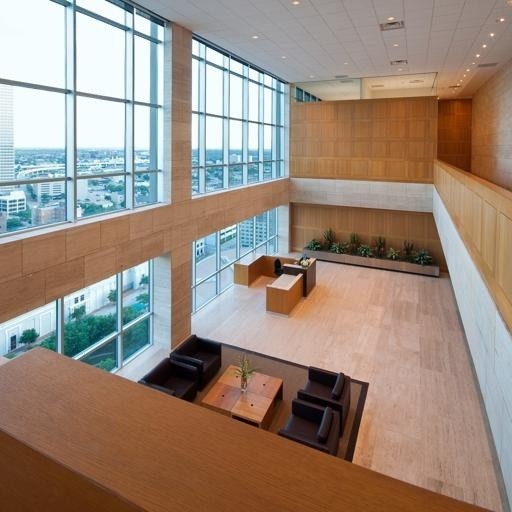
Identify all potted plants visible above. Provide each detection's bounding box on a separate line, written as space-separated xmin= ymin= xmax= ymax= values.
xmin=302 ymin=227 xmax=441 ymax=278
xmin=227 ymin=349 xmax=268 ymax=392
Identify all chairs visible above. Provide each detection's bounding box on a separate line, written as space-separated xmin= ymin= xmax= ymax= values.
xmin=135 ymin=330 xmax=224 ymax=403
xmin=272 ymin=256 xmax=286 ymax=277
xmin=280 ymin=364 xmax=352 ymax=457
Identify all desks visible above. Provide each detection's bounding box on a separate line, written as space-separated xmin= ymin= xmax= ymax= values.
xmin=265 ymin=274 xmax=303 ymax=316
xmin=234 ymin=251 xmax=300 ymax=287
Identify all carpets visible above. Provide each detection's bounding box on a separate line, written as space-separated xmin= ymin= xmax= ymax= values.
xmin=190 ymin=342 xmax=369 ymax=465
